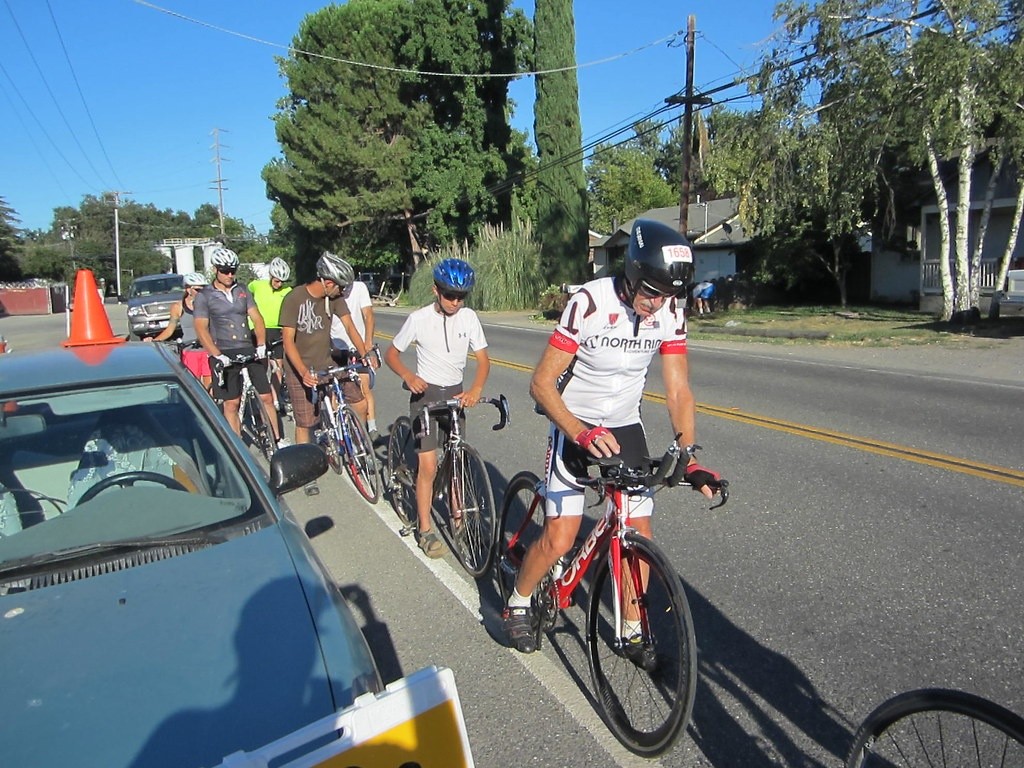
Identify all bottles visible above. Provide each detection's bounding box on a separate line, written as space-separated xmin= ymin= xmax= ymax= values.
xmin=552 ymin=545 xmax=579 ymax=579
xmin=435 ymin=446 xmax=446 ymax=466
xmin=318 ymin=391 xmax=329 ymax=428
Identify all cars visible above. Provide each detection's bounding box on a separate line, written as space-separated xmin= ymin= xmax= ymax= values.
xmin=353 ymin=273 xmax=380 ymax=294
xmin=1 ymin=340 xmax=389 ymax=768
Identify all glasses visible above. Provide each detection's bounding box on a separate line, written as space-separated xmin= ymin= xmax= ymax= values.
xmin=334 ymin=284 xmax=344 ymax=292
xmin=219 ymin=268 xmax=236 ymax=275
xmin=191 ymin=288 xmax=200 ymax=292
xmin=639 ymin=280 xmax=678 ymax=298
xmin=440 ymin=292 xmax=467 ymax=300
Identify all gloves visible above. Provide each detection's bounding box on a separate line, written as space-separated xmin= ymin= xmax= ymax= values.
xmin=253 ymin=344 xmax=267 ymax=361
xmin=211 ymin=354 xmax=232 ymax=368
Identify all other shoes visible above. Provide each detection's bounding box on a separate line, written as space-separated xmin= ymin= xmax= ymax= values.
xmin=269 ymin=438 xmax=290 ymax=455
xmin=303 ymin=480 xmax=320 ymax=495
xmin=367 ymin=429 xmax=388 ymax=459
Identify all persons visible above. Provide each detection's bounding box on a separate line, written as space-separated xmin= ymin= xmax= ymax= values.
xmin=96 ymin=271 xmax=106 ymax=304
xmin=689 ymin=282 xmax=716 ymax=314
xmin=277 ymin=251 xmax=383 ymax=496
xmin=383 ymin=259 xmax=489 ymax=559
xmin=330 ymin=281 xmax=383 ymax=445
xmin=193 ymin=248 xmax=292 ymax=450
xmin=502 ymin=220 xmax=720 ymax=679
xmin=247 ymin=257 xmax=293 ymax=421
xmin=143 ymin=273 xmax=212 ymax=399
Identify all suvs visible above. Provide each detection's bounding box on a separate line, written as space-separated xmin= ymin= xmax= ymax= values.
xmin=123 ymin=272 xmax=185 ymax=341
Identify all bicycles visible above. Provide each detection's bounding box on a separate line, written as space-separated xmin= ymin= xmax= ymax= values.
xmin=842 ymin=684 xmax=1024 ymax=768
xmin=214 ymin=336 xmax=284 ymax=469
xmin=385 ymin=373 xmax=512 ymax=580
xmin=493 ymin=428 xmax=732 ymax=759
xmin=305 ymin=350 xmax=382 ymax=505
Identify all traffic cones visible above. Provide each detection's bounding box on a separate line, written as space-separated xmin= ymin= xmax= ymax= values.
xmin=56 ymin=268 xmax=126 ymax=349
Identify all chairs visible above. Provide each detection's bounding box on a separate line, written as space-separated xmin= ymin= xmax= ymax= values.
xmin=64 ymin=405 xmax=210 ymax=511
xmin=140 ymin=284 xmax=151 ymax=295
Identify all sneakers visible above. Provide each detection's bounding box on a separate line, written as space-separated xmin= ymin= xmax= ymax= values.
xmin=503 ymin=595 xmax=536 ymax=653
xmin=418 ymin=528 xmax=450 ymax=560
xmin=621 ymin=635 xmax=674 ymax=675
xmin=453 ymin=520 xmax=472 ymax=561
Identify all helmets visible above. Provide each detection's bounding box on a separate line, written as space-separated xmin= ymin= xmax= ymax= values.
xmin=269 ymin=257 xmax=290 ymax=281
xmin=433 ymin=258 xmax=475 ymax=293
xmin=316 ymin=251 xmax=354 ymax=287
xmin=183 ymin=272 xmax=209 ymax=287
xmin=625 ymin=218 xmax=694 ymax=291
xmin=211 ymin=248 xmax=240 ymax=269
